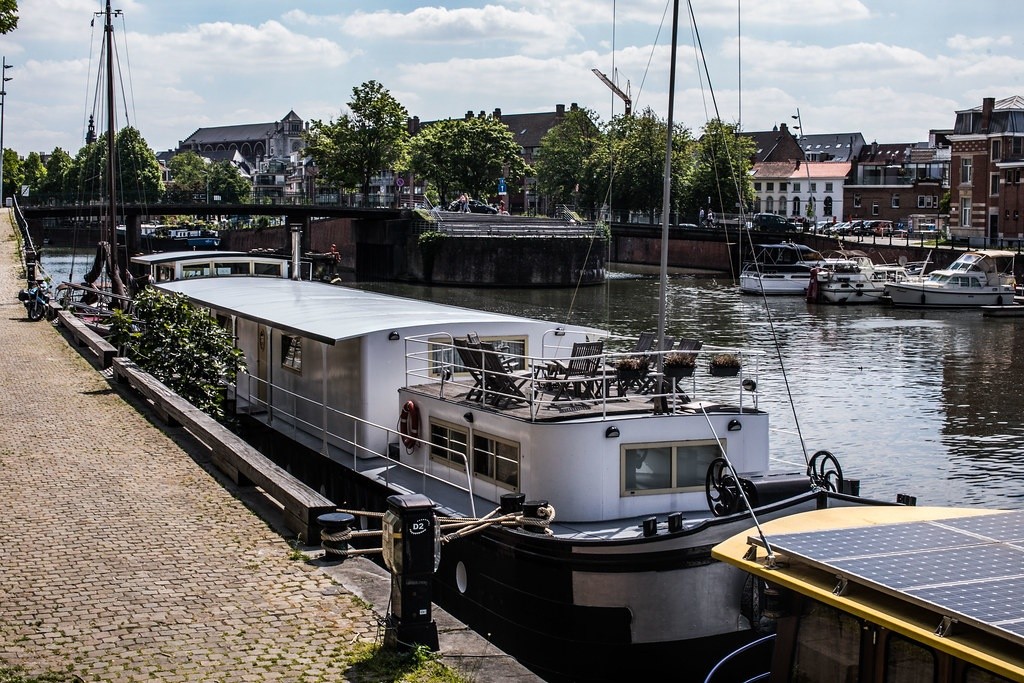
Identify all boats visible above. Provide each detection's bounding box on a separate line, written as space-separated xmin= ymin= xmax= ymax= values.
xmin=710 ymin=503 xmax=1024 ymax=683
xmin=109 ymin=222 xmax=225 ymax=270
xmin=812 ymin=249 xmax=934 ymax=306
xmin=57 ymin=280 xmax=129 ymax=325
xmin=738 ymin=240 xmax=868 ymax=296
xmin=131 ymin=0 xmax=912 ymax=658
xmin=884 ymin=249 xmax=1024 ymax=309
xmin=979 ymin=305 xmax=1024 ymax=318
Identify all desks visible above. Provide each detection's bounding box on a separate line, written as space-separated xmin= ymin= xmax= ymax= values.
xmin=528 ymin=362 xmax=617 ymax=390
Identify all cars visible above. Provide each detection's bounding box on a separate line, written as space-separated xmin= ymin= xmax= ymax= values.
xmin=893 ymin=228 xmax=907 ymax=236
xmin=448 ymin=200 xmax=497 ymax=215
xmin=807 ymin=218 xmax=894 ymax=237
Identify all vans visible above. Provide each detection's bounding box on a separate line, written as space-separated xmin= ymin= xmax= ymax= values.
xmin=752 ymin=214 xmax=797 ymax=232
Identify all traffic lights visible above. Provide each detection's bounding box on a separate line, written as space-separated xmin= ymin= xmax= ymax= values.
xmin=911 ymin=177 xmax=918 ymax=188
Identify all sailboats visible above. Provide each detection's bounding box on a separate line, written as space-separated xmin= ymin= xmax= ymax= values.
xmin=64 ymin=0 xmax=155 ymax=341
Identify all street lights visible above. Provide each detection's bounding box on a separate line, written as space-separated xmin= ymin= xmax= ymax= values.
xmin=0 ymin=64 xmax=14 ymax=207
xmin=792 ymin=115 xmax=816 ymax=223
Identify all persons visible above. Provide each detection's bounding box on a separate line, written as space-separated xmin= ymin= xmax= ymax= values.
xmin=699 ymin=207 xmax=704 ymax=225
xmin=707 ymin=208 xmax=713 ymax=228
xmin=460 ymin=193 xmax=470 ymax=213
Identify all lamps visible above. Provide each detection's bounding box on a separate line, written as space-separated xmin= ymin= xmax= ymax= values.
xmin=742 ymin=379 xmax=756 ymax=391
xmin=606 ymin=426 xmax=620 ymax=438
xmin=554 ymin=328 xmax=565 ymax=336
xmin=463 ymin=412 xmax=473 ymax=423
xmin=727 ymin=420 xmax=741 ymax=431
xmin=389 ymin=331 xmax=400 ymax=340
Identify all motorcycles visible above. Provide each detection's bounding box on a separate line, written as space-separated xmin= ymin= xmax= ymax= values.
xmin=18 ymin=278 xmax=52 ymax=322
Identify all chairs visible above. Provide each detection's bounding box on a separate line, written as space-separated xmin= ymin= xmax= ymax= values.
xmin=453 ymin=332 xmax=703 ymax=409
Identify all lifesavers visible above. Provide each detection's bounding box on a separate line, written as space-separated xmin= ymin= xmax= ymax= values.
xmin=399 ymin=400 xmax=419 ymax=447
